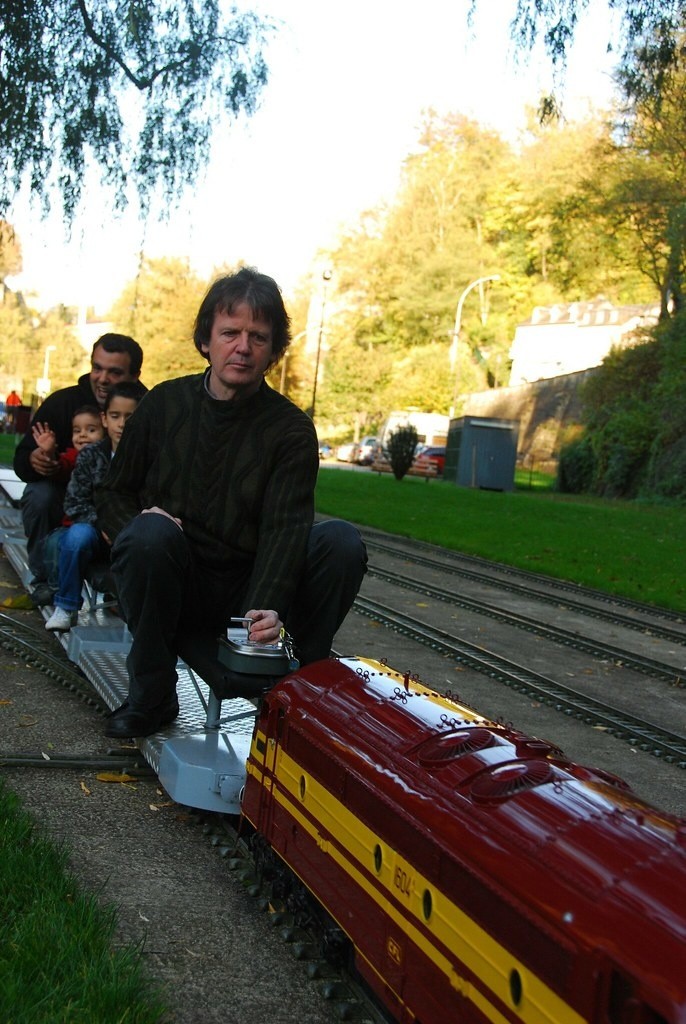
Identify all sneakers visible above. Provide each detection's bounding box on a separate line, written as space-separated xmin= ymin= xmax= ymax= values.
xmin=45 ymin=606 xmax=78 ymax=632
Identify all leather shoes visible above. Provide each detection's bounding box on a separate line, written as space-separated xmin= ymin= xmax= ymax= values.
xmin=104 ymin=695 xmax=180 ymax=739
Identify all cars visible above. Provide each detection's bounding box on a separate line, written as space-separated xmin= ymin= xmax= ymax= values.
xmin=316 ymin=436 xmax=378 ymax=465
xmin=411 ymin=445 xmax=447 ymax=475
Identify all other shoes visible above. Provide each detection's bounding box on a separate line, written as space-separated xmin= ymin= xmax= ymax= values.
xmin=102 ymin=593 xmax=122 ymax=618
xmin=30 ymin=574 xmax=57 ymax=601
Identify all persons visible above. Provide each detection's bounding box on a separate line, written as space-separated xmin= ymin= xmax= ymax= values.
xmin=95 ymin=268 xmax=369 ymax=738
xmin=0 ymin=332 xmax=151 ymax=630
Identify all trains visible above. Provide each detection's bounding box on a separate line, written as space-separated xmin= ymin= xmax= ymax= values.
xmin=0 ymin=469 xmax=685 ymax=1024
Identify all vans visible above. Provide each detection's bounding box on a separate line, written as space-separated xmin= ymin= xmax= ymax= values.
xmin=380 ymin=411 xmax=451 ymax=465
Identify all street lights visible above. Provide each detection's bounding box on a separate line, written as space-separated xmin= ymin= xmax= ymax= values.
xmin=447 ymin=274 xmax=502 ymax=418
xmin=308 ymin=265 xmax=333 ymax=419
xmin=38 ymin=345 xmax=55 ymax=409
